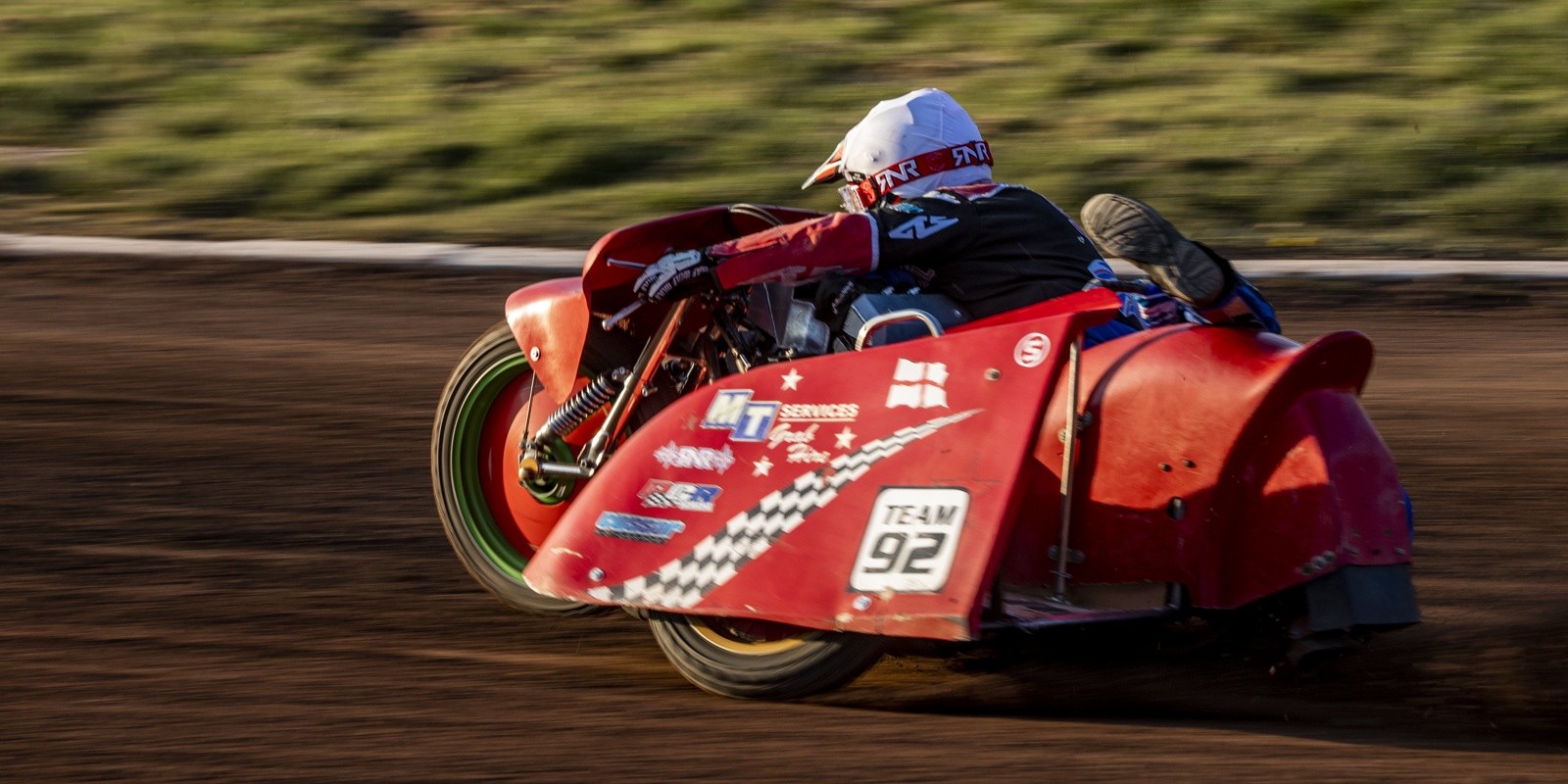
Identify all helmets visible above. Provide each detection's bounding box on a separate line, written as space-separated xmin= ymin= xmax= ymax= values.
xmin=801 ymin=87 xmax=993 ymax=213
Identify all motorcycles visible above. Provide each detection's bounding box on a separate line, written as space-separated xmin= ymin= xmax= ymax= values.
xmin=432 ymin=203 xmax=1424 ymax=701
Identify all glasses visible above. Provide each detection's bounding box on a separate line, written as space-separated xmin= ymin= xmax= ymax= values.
xmin=837 ymin=184 xmax=868 ymax=213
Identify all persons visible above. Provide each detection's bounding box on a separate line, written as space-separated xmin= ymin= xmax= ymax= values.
xmin=629 ymin=84 xmax=1146 ymax=367
xmin=1066 ymin=191 xmax=1284 ymax=341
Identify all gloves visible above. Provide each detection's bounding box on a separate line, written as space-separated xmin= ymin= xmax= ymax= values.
xmin=632 ymin=248 xmax=712 ymax=304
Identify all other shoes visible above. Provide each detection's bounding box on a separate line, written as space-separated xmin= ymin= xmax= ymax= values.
xmin=1080 ymin=193 xmax=1233 ymax=306
